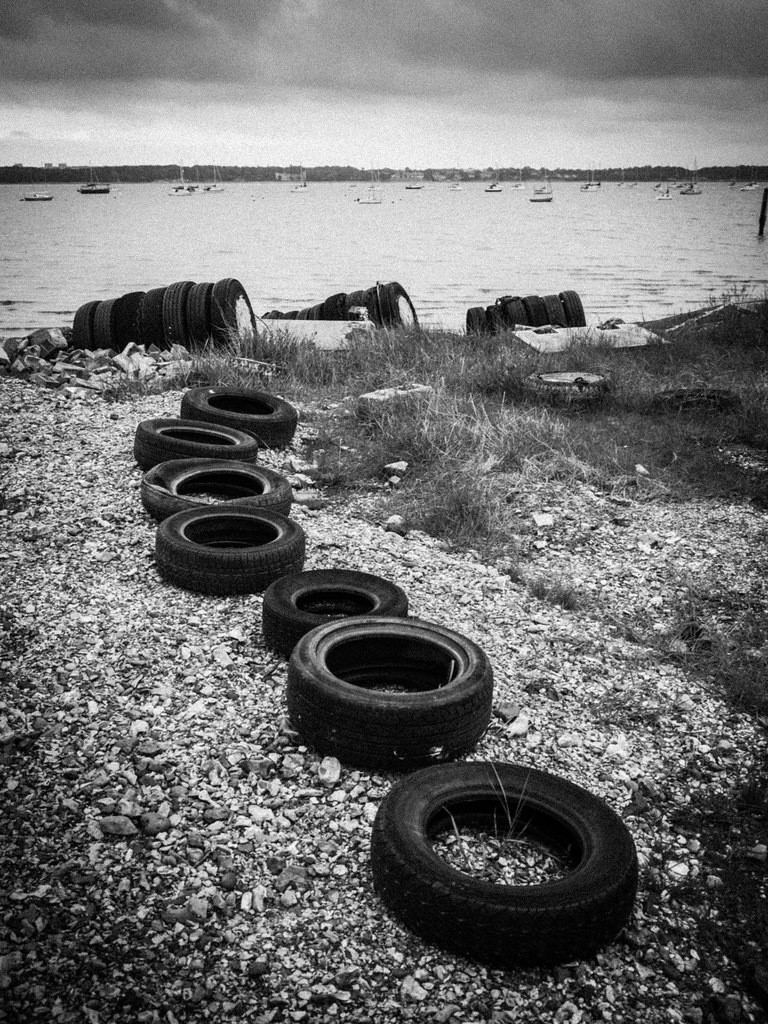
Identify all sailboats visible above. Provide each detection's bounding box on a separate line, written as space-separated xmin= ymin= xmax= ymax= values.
xmin=511 ymin=159 xmax=761 ymax=200
xmin=291 ymin=157 xmax=311 ymax=193
xmin=350 ymin=161 xmax=384 ymax=205
xmin=78 ymin=159 xmax=111 ymax=193
xmin=168 ymin=153 xmax=224 ymax=197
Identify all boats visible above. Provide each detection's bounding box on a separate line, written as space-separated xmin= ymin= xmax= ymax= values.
xmin=406 ymin=184 xmax=422 ymax=190
xmin=21 ymin=191 xmax=53 ymax=202
xmin=531 ymin=198 xmax=553 ymax=203
xmin=485 ymin=186 xmax=502 ymax=192
